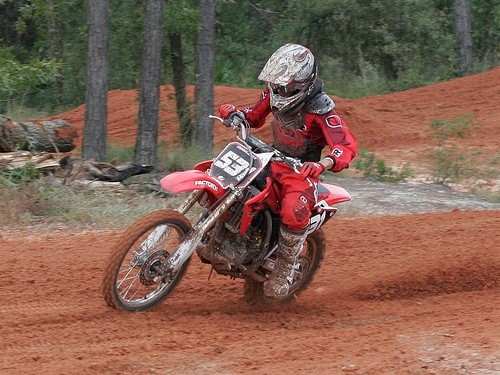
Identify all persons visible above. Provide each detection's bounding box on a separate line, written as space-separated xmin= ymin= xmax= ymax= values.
xmin=219 ymin=43 xmax=357 ymax=296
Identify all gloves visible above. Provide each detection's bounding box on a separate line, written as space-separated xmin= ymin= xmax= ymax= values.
xmin=299 ymin=162 xmax=325 ymax=181
xmin=218 ymin=103 xmax=238 ymax=119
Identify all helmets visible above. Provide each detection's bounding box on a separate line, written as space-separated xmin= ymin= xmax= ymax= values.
xmin=258 ymin=43 xmax=318 ymax=116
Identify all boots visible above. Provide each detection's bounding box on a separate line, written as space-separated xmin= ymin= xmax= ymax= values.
xmin=264 ymin=224 xmax=309 ymax=299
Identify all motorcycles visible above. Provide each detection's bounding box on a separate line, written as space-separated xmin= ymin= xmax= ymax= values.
xmin=100 ymin=110 xmax=353 ymax=310
